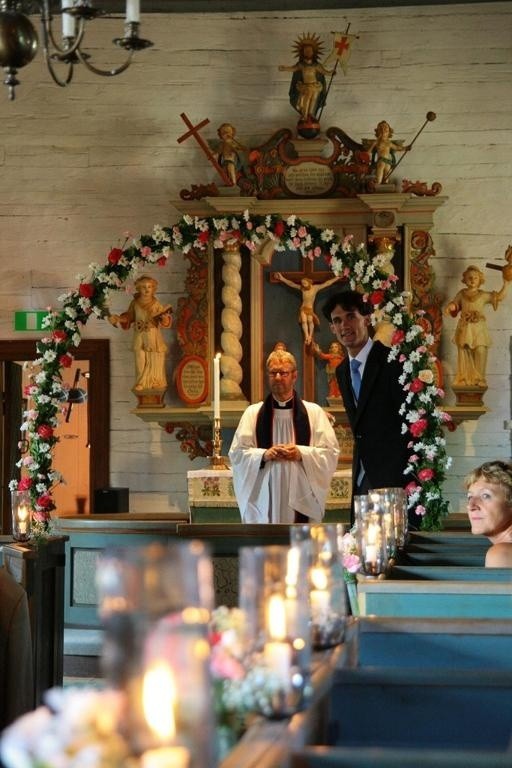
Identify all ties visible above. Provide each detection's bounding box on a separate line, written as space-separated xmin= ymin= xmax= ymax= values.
xmin=349 ymin=357 xmax=366 ymax=403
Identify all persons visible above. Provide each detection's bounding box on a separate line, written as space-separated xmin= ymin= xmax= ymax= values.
xmin=309 ymin=338 xmax=345 ymax=399
xmin=364 ymin=120 xmax=412 ymax=186
xmin=321 ymin=290 xmax=423 ymax=536
xmin=277 ymin=43 xmax=337 ymax=122
xmin=209 ymin=122 xmax=248 ymax=187
xmin=107 ymin=272 xmax=175 ymax=391
xmin=274 ymin=271 xmax=344 ymax=345
xmin=460 ymin=459 xmax=511 ymax=569
xmin=227 ymin=343 xmax=341 ymax=524
xmin=445 ymin=265 xmax=512 ymax=389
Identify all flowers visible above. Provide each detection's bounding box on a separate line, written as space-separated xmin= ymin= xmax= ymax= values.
xmin=8 ymin=207 xmax=454 ymax=543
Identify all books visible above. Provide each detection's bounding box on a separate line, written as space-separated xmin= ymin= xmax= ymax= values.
xmin=151 ymin=305 xmax=174 ymax=325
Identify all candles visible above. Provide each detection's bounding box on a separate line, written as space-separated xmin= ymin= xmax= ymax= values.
xmin=140 ymin=743 xmax=190 ymax=767
xmin=263 ymin=641 xmax=293 ymax=688
xmin=16 ymin=506 xmax=28 ymax=534
xmin=214 ymin=352 xmax=221 ymax=418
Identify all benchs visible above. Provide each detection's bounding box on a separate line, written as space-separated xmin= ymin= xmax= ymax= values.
xmin=295 ymin=531 xmax=512 ymax=768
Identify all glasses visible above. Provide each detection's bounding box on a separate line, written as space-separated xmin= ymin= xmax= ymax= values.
xmin=267 ymin=370 xmax=294 ymax=377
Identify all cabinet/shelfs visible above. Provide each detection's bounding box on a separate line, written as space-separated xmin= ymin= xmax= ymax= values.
xmin=57 ymin=514 xmax=295 ymax=687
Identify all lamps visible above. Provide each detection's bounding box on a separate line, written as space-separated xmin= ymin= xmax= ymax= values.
xmin=0 ymin=0 xmax=155 ymax=100
